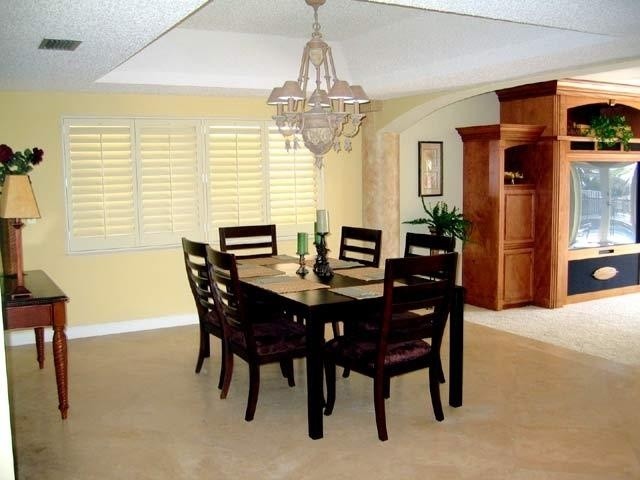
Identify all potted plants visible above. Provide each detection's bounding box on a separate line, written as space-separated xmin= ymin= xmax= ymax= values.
xmin=573 ymin=115 xmax=632 ymax=152
xmin=401 ymin=195 xmax=473 ymax=256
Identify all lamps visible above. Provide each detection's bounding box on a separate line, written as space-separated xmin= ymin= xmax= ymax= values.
xmin=0 ymin=174 xmax=41 ymax=300
xmin=265 ymin=0 xmax=370 ymax=171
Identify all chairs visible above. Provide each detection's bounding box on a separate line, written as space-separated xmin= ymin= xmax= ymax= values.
xmin=219 ymin=224 xmax=294 ymax=323
xmin=322 ymin=252 xmax=456 ymax=441
xmin=342 ymin=233 xmax=456 ymax=382
xmin=205 ymin=244 xmax=327 ymax=421
xmin=181 ymin=238 xmax=288 ymax=389
xmin=298 ymin=226 xmax=383 ymax=336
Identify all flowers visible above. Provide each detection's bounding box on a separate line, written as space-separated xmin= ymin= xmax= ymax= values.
xmin=0 ymin=144 xmax=44 ymax=187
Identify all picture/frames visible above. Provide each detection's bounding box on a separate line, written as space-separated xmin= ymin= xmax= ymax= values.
xmin=417 ymin=140 xmax=443 ymax=196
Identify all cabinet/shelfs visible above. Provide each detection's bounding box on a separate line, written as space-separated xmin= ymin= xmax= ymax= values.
xmin=456 ymin=122 xmax=548 ymax=311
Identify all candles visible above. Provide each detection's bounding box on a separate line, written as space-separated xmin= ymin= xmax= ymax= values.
xmin=316 ymin=209 xmax=329 ymax=232
xmin=298 ymin=233 xmax=308 ymax=255
xmin=314 ymin=223 xmax=321 ymax=243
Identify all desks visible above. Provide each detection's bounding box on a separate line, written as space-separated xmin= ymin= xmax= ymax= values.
xmin=0 ymin=269 xmax=70 ymax=420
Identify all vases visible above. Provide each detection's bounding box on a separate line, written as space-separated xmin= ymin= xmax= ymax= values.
xmin=0 ymin=217 xmax=18 ymax=274
